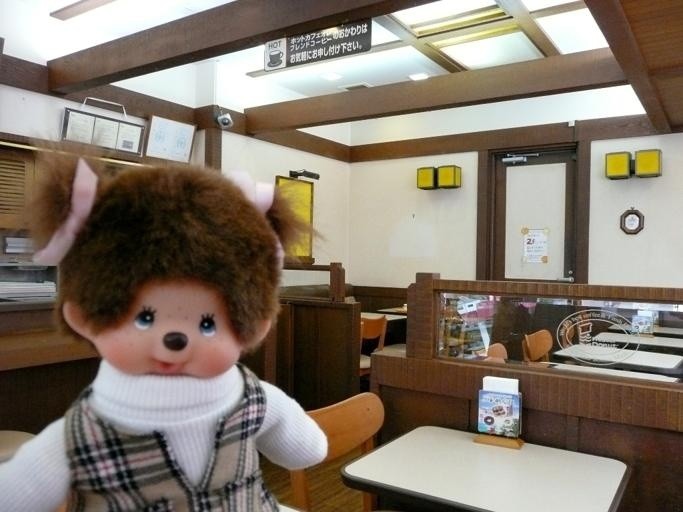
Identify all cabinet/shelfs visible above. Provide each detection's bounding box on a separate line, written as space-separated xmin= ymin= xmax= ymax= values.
xmin=0 ymin=141 xmax=68 ymax=338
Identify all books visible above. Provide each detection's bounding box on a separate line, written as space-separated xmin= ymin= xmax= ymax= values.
xmin=0 ymin=280 xmax=58 ymax=304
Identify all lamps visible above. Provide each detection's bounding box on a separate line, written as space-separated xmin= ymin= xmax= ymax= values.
xmin=604 ymin=148 xmax=662 ymax=179
xmin=416 ymin=164 xmax=461 ymax=191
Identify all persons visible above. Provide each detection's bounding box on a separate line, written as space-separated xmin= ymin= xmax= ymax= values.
xmin=0 ymin=136 xmax=327 ymax=511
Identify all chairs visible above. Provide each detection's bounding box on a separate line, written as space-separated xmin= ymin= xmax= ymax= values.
xmin=278 ymin=390 xmax=378 ymax=512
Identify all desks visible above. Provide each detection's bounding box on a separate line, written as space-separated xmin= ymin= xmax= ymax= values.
xmin=336 ymin=416 xmax=635 ymax=510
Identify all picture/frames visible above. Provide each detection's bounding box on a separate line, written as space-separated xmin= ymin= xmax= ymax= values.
xmin=274 ymin=174 xmax=316 ymax=266
